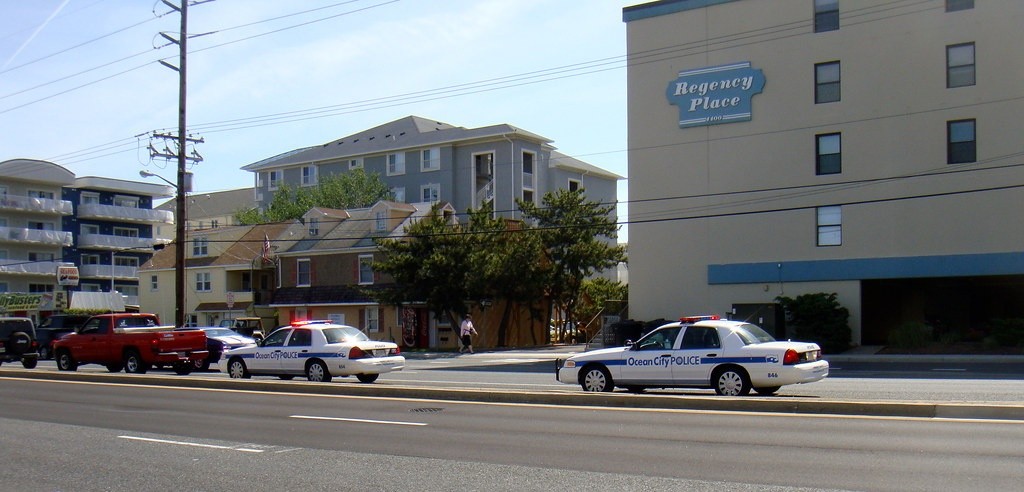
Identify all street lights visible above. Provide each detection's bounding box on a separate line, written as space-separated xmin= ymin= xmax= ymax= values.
xmin=139 ymin=171 xmax=186 ymax=328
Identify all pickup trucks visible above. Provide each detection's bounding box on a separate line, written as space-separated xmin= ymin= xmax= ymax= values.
xmin=54 ymin=313 xmax=209 ymax=374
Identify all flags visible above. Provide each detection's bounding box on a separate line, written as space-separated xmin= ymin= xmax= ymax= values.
xmin=261 ymin=234 xmax=270 ymax=253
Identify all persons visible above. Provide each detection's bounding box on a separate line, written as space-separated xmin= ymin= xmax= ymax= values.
xmin=459 ymin=314 xmax=478 ymax=354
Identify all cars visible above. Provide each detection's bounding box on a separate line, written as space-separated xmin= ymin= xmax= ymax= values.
xmin=174 ymin=327 xmax=256 ymax=359
xmin=218 ymin=320 xmax=405 ymax=383
xmin=36 ymin=313 xmax=96 ymax=359
xmin=554 ymin=316 xmax=829 ymax=395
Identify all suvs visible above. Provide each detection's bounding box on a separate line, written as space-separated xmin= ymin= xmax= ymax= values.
xmin=1 ymin=317 xmax=40 ymax=369
xmin=219 ymin=317 xmax=266 ymax=344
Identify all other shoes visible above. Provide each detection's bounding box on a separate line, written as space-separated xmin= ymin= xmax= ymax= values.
xmin=458 ymin=350 xmax=463 ymax=354
xmin=470 ymin=352 xmax=475 ymax=354
